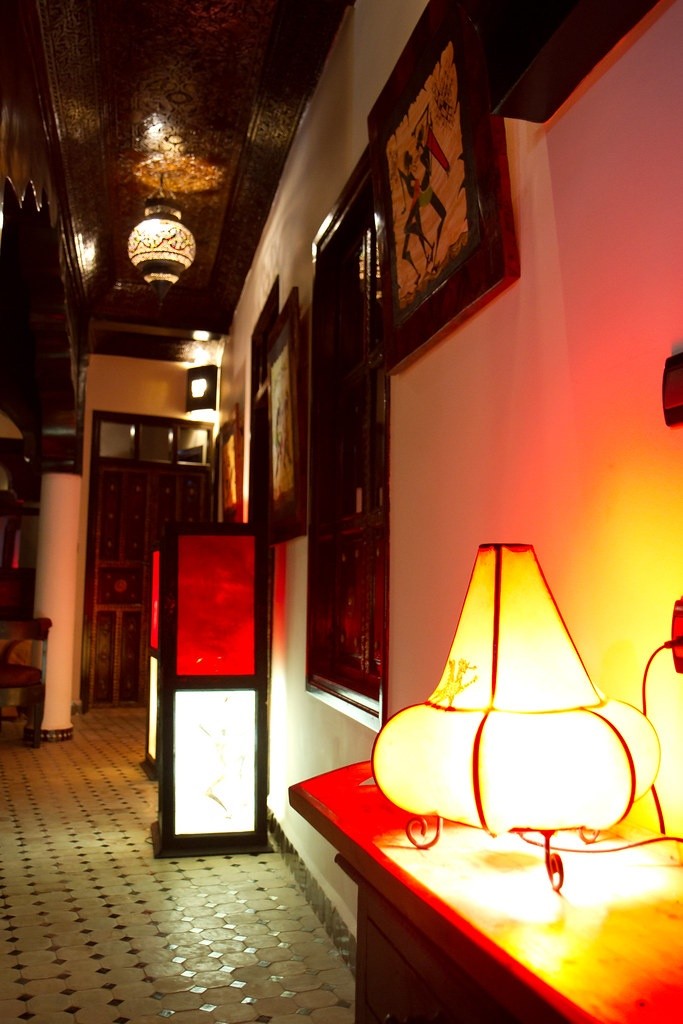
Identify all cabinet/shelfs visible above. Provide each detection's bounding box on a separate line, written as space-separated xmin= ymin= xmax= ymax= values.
xmin=288 ymin=760 xmax=683 ymax=1024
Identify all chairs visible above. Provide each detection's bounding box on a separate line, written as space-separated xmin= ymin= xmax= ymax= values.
xmin=0 ymin=616 xmax=53 ymax=748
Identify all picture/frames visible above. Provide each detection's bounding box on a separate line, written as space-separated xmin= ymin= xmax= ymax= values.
xmin=265 ymin=287 xmax=306 ymax=548
xmin=478 ymin=0 xmax=661 ymax=122
xmin=366 ymin=0 xmax=521 ymax=376
xmin=220 ymin=401 xmax=243 ymax=523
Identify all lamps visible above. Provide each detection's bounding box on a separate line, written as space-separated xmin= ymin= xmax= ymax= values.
xmin=127 ymin=173 xmax=197 ymax=307
xmin=370 ymin=540 xmax=661 ymax=892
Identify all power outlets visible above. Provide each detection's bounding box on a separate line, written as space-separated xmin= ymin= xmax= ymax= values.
xmin=670 ymin=600 xmax=683 ymax=674
